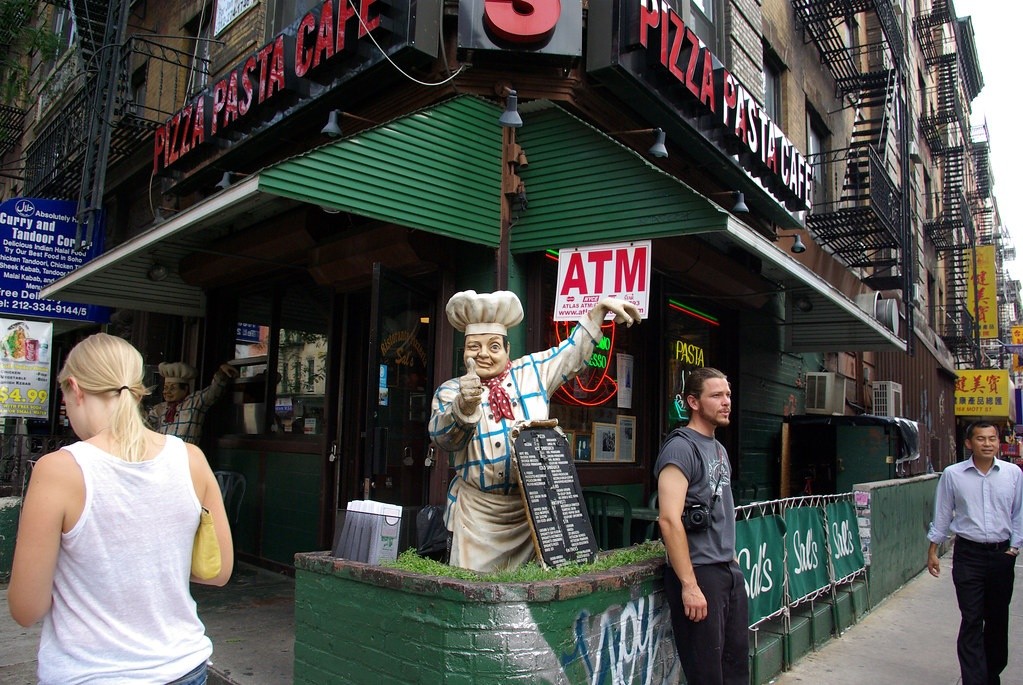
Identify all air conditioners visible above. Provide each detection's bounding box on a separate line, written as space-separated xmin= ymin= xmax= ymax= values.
xmin=909 ymin=141 xmax=923 ymax=164
xmin=805 ymin=372 xmax=847 ymax=416
xmin=892 ymin=0 xmax=903 ymax=15
xmin=913 ymin=283 xmax=921 ymax=306
xmin=871 ymin=381 xmax=903 ymax=418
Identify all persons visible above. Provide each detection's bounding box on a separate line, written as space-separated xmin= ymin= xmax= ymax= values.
xmin=926 ymin=419 xmax=1023 ymax=685
xmin=139 ymin=361 xmax=238 ymax=446
xmin=7 ymin=332 xmax=234 ymax=685
xmin=427 ymin=289 xmax=642 ymax=573
xmin=654 ymin=367 xmax=749 ymax=685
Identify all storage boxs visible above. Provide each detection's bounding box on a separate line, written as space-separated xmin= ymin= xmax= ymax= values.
xmin=240 ymin=364 xmax=267 ymax=378
xmin=304 ymin=417 xmax=321 ymax=434
xmin=331 ymin=508 xmax=402 ymax=567
xmin=243 ymin=402 xmax=265 ymax=435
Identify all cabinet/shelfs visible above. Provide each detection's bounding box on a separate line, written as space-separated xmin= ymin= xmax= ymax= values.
xmin=226 ymin=355 xmax=268 ymax=384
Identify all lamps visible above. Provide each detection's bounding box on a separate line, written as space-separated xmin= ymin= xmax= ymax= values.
xmin=152 ymin=206 xmax=180 ymax=227
xmin=606 ymin=127 xmax=670 ymax=158
xmin=146 ymin=261 xmax=169 ymax=282
xmin=494 ymin=78 xmax=524 ymax=128
xmin=795 ymin=292 xmax=813 ymax=313
xmin=320 ymin=109 xmax=377 ymax=138
xmin=773 ymin=232 xmax=807 ymax=254
xmin=213 ymin=171 xmax=251 ymax=190
xmin=707 ymin=190 xmax=750 ymax=213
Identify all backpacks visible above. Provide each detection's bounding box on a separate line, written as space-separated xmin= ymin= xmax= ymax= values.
xmin=417 ymin=505 xmax=453 ymax=565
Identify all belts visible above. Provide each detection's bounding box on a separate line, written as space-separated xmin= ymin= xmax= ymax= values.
xmin=955 ymin=535 xmax=1010 ymax=550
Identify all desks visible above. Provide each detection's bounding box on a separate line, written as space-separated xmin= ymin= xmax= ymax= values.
xmin=591 ymin=507 xmax=661 ymax=522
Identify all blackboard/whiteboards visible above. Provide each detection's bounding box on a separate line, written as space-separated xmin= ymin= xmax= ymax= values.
xmin=512 ymin=425 xmax=600 ymax=567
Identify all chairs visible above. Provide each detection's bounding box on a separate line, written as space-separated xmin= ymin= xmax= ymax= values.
xmin=646 ymin=489 xmax=663 ymax=542
xmin=582 ymin=490 xmax=632 ymax=552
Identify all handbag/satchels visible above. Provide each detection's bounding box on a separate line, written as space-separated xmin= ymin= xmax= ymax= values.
xmin=191 ymin=506 xmax=222 ymax=581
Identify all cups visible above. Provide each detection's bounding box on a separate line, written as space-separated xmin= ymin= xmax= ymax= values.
xmin=616 ymin=352 xmax=633 ymax=408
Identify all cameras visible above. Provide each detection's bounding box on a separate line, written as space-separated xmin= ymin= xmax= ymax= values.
xmin=681 ymin=502 xmax=711 ymax=531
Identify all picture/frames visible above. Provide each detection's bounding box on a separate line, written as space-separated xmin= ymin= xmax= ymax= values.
xmin=572 ymin=431 xmax=593 ymax=462
xmin=615 ymin=414 xmax=636 ymax=463
xmin=564 ymin=430 xmax=576 ymax=457
xmin=591 ymin=422 xmax=616 ymax=462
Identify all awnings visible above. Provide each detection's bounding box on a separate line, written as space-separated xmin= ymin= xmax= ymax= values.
xmin=509 ymin=98 xmax=907 ymax=352
xmin=38 ymin=91 xmax=507 ymax=319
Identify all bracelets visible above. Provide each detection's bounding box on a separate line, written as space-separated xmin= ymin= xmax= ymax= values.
xmin=1007 ymin=547 xmax=1020 ymax=555
